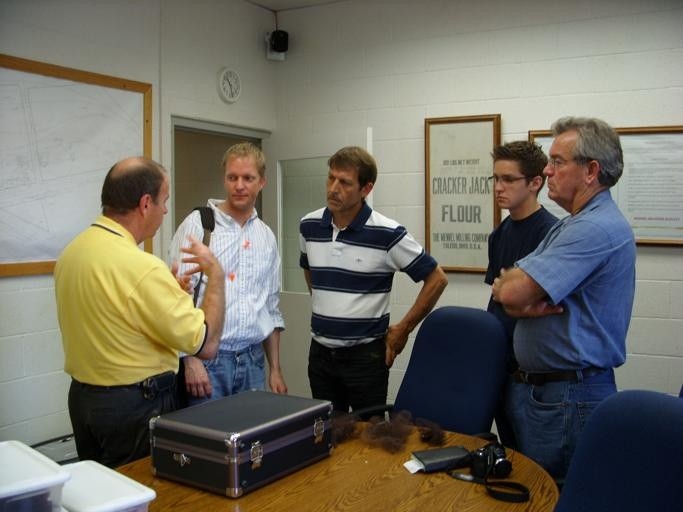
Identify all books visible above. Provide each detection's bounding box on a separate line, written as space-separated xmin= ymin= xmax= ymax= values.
xmin=411 ymin=446 xmax=469 ymax=473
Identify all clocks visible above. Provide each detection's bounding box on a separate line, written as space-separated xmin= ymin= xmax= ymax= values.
xmin=218 ymin=65 xmax=241 ymax=103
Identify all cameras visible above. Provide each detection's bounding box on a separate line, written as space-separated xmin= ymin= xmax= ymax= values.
xmin=472 ymin=442 xmax=512 ymax=478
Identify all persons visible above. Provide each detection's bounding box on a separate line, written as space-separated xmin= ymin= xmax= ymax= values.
xmin=483 ymin=141 xmax=560 ymax=447
xmin=53 ymin=155 xmax=227 ymax=470
xmin=297 ymin=146 xmax=448 ymax=423
xmin=492 ymin=114 xmax=637 ymax=490
xmin=168 ymin=142 xmax=289 ymax=407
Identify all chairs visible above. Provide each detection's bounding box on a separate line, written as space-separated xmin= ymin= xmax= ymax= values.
xmin=339 ymin=305 xmax=503 ymax=438
xmin=558 ymin=391 xmax=681 ymax=510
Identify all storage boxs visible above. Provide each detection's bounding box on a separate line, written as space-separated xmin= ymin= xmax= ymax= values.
xmin=149 ymin=391 xmax=333 ymax=500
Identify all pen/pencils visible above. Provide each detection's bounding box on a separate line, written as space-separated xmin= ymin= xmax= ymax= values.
xmin=447 ymin=469 xmax=473 ymax=482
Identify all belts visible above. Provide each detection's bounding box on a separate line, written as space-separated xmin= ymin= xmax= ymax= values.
xmin=513 ymin=367 xmax=604 ymax=385
xmin=71 ymin=380 xmax=144 ymax=391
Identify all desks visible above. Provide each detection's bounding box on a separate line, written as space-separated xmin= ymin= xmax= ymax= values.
xmin=115 ymin=422 xmax=559 ymax=511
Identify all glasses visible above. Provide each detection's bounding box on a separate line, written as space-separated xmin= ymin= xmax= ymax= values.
xmin=547 ymin=157 xmax=590 ymax=170
xmin=487 ymin=175 xmax=535 ymax=184
